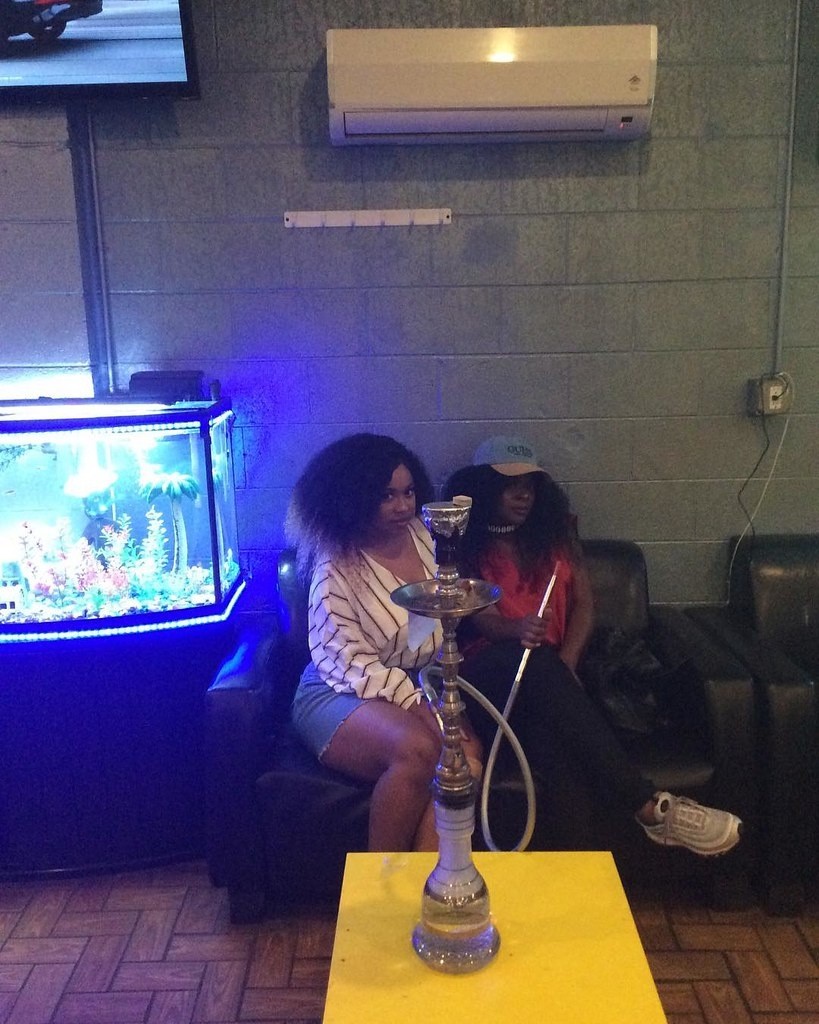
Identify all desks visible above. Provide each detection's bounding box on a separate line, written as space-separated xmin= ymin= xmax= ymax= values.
xmin=321 ymin=850 xmax=668 ymax=1024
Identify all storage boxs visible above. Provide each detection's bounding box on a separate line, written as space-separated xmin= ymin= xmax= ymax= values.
xmin=0 ymin=396 xmax=250 ymax=647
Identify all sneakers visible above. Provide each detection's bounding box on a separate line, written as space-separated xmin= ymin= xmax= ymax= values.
xmin=635 ymin=791 xmax=742 ymax=857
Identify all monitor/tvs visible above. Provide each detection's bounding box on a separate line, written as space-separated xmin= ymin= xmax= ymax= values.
xmin=0 ymin=0 xmax=201 ymax=106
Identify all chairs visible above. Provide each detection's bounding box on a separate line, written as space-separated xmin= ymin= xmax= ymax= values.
xmin=201 ymin=531 xmax=758 ymax=924
xmin=683 ymin=532 xmax=818 ymax=917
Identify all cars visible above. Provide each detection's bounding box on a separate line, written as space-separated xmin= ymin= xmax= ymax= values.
xmin=0 ymin=0 xmax=104 ymax=47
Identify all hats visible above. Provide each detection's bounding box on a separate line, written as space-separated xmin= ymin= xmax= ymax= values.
xmin=473 ymin=435 xmax=552 ymax=479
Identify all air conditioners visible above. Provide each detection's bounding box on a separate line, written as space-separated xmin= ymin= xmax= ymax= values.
xmin=324 ymin=25 xmax=660 ymax=147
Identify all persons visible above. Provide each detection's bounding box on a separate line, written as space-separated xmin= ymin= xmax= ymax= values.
xmin=282 ymin=432 xmax=484 ymax=851
xmin=437 ymin=433 xmax=752 ymax=858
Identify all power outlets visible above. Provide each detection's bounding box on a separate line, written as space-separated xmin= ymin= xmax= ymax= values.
xmin=770 ymin=384 xmax=782 ymax=410
